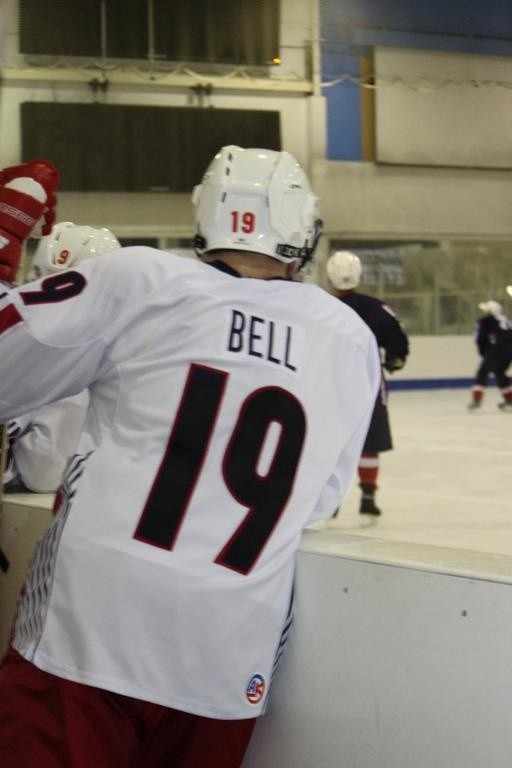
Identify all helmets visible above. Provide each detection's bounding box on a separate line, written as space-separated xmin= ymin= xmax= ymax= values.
xmin=326 ymin=251 xmax=362 ymax=291
xmin=26 ymin=222 xmax=122 ymax=284
xmin=190 ymin=144 xmax=323 ymax=264
xmin=478 ymin=300 xmax=503 ymax=315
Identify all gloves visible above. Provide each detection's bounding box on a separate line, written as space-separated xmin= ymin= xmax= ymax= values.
xmin=1 ymin=161 xmax=57 ymax=281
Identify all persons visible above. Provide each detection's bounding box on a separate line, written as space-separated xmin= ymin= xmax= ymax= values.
xmin=0 ymin=142 xmax=381 ymax=768
xmin=321 ymin=247 xmax=411 ymax=517
xmin=0 ymin=218 xmax=125 ymax=494
xmin=471 ymin=300 xmax=512 ymax=411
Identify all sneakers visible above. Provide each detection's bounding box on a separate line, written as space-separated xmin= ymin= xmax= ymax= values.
xmin=467 ymin=399 xmax=482 ymax=410
xmin=499 ymin=402 xmax=512 ymax=412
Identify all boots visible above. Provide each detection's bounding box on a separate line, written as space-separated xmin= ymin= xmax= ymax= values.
xmin=358 ymin=483 xmax=380 ymax=514
